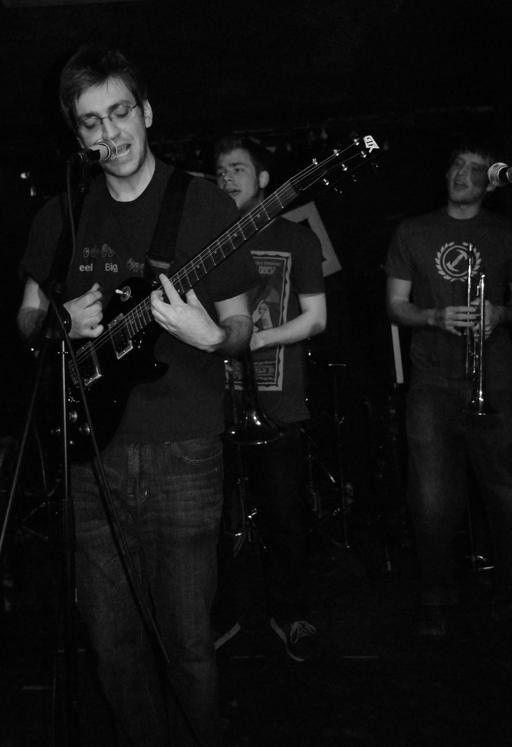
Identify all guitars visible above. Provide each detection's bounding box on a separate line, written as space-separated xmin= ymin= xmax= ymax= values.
xmin=22 ymin=133 xmax=388 ymax=464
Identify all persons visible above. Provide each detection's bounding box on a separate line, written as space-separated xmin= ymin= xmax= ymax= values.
xmin=213 ymin=131 xmax=330 ymax=665
xmin=11 ymin=37 xmax=266 ymax=747
xmin=381 ymin=142 xmax=512 ymax=640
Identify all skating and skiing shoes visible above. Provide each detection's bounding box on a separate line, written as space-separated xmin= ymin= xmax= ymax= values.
xmin=418 ymin=603 xmax=449 ymax=637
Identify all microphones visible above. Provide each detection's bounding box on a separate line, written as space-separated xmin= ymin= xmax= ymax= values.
xmin=488 ymin=162 xmax=512 ymax=187
xmin=69 ymin=138 xmax=117 ymax=168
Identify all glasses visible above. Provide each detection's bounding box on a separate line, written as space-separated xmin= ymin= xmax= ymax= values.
xmin=75 ymin=100 xmax=142 ymax=133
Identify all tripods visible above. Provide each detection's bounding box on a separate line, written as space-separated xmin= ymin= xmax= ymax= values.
xmin=308 ymin=358 xmax=386 ymax=553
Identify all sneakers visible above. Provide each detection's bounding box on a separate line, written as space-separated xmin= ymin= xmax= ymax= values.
xmin=268 ymin=613 xmax=322 ymax=665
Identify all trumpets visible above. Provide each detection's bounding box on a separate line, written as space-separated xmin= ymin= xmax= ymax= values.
xmin=467 ymin=244 xmax=490 ymax=417
